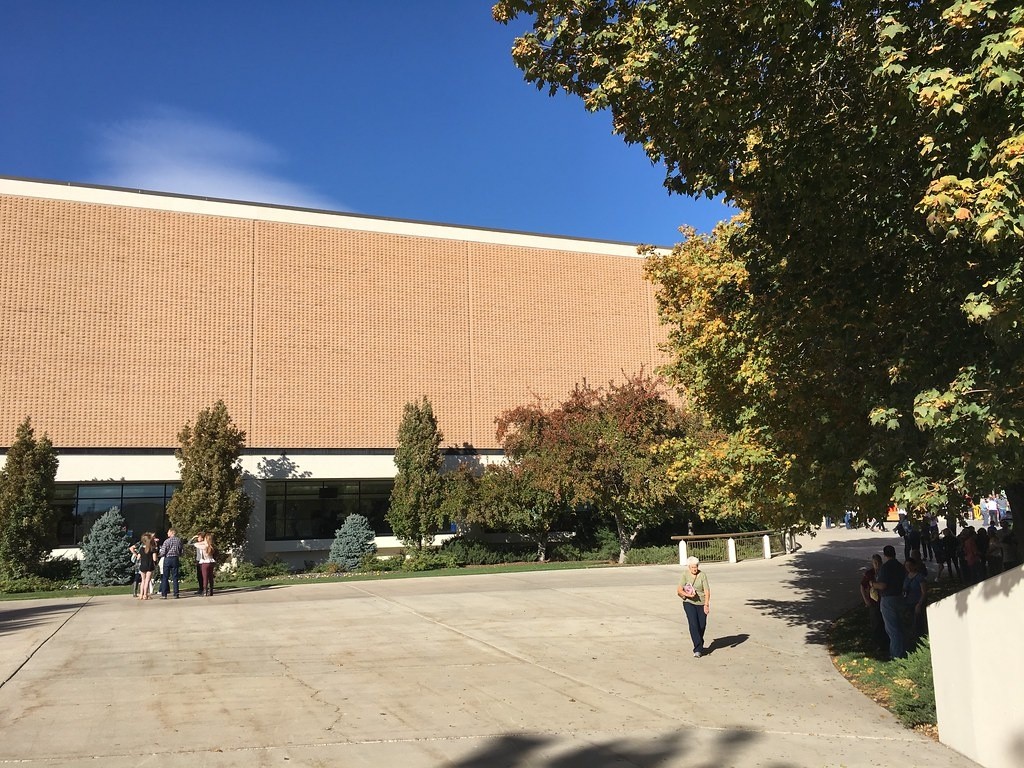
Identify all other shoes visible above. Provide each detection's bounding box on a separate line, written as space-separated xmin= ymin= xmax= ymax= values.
xmin=159 ymin=596 xmax=167 ymax=599
xmin=141 ymin=595 xmax=152 ymax=600
xmin=194 ymin=591 xmax=201 ymax=594
xmin=694 ymin=651 xmax=702 ymax=657
xmin=174 ymin=596 xmax=178 ymax=599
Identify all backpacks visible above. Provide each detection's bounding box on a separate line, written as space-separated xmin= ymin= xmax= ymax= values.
xmin=898 ymin=523 xmax=908 ymax=537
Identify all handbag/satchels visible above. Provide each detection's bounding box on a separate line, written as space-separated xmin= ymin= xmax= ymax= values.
xmin=131 ymin=555 xmax=137 ymax=562
xmin=870 ymin=586 xmax=878 ymax=601
xmin=678 ymin=593 xmax=686 ymax=600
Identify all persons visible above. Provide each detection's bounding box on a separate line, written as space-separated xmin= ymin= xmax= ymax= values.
xmin=845 ymin=510 xmax=888 ymax=532
xmin=157 ymin=556 xmax=170 ymax=595
xmin=826 ymin=515 xmax=831 ymax=528
xmin=909 ymin=549 xmax=928 ymax=577
xmin=979 ymin=494 xmax=1006 ymax=526
xmin=901 ymin=515 xmax=939 ymax=561
xmin=861 ymin=554 xmax=882 ymax=637
xmin=869 ymin=545 xmax=906 ymax=660
xmin=677 ymin=557 xmax=710 ymax=659
xmin=130 ymin=532 xmax=157 ymax=602
xmin=158 ymin=528 xmax=183 ymax=599
xmin=187 ymin=532 xmax=216 ymax=597
xmin=931 ymin=520 xmax=1012 ymax=584
xmin=902 ymin=558 xmax=926 ymax=636
